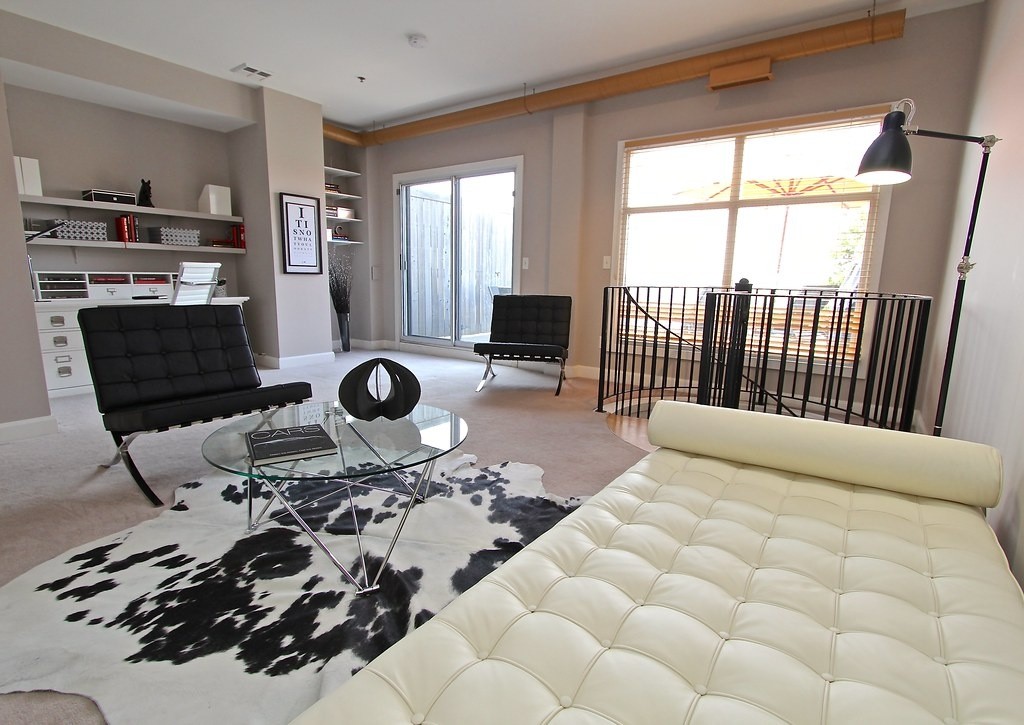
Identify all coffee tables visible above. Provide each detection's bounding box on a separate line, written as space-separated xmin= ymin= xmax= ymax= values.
xmin=204 ymin=399 xmax=470 ymax=595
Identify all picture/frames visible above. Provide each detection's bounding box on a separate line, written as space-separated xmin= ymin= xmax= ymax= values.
xmin=279 ymin=192 xmax=324 ymax=275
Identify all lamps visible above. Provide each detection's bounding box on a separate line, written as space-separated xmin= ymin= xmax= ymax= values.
xmin=856 ymin=97 xmax=1003 ymax=438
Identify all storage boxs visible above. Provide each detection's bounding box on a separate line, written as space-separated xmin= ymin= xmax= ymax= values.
xmin=337 ymin=206 xmax=356 ymax=219
xmin=198 ymin=184 xmax=233 ymax=216
xmin=81 ymin=188 xmax=138 ymax=207
xmin=13 ymin=156 xmax=43 ymax=197
xmin=148 ymin=226 xmax=201 ymax=247
xmin=327 ymin=229 xmax=333 ymax=240
xmin=47 ymin=218 xmax=109 ymax=241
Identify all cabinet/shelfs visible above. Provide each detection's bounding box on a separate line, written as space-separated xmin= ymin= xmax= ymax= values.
xmin=33 ymin=270 xmax=179 ymax=300
xmin=34 ymin=296 xmax=250 ymax=399
xmin=19 ymin=193 xmax=246 ymax=254
xmin=324 ymin=166 xmax=365 ymax=246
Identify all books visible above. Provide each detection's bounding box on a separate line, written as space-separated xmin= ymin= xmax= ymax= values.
xmin=246 ymin=424 xmax=338 ymax=467
xmin=90 ymin=277 xmax=128 ymax=283
xmin=332 ymin=234 xmax=349 ymax=241
xmin=134 ymin=276 xmax=166 ymax=283
xmin=207 ymin=224 xmax=245 ymax=248
xmin=325 ymin=182 xmax=340 ymax=193
xmin=326 ymin=206 xmax=338 ymax=217
xmin=115 ymin=214 xmax=139 ymax=242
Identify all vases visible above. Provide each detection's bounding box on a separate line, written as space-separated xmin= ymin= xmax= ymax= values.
xmin=337 ymin=313 xmax=351 ymax=352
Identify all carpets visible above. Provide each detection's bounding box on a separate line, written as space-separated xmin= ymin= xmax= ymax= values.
xmin=0 ymin=447 xmax=593 ymax=725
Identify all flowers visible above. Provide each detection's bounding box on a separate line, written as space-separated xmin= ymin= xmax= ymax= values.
xmin=327 ymin=244 xmax=355 ymax=313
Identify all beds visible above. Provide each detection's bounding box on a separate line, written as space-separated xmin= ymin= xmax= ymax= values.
xmin=291 ymin=400 xmax=1024 ymax=725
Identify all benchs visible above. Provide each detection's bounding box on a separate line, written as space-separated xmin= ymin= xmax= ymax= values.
xmin=474 ymin=294 xmax=573 ymax=397
xmin=75 ymin=303 xmax=312 ymax=507
